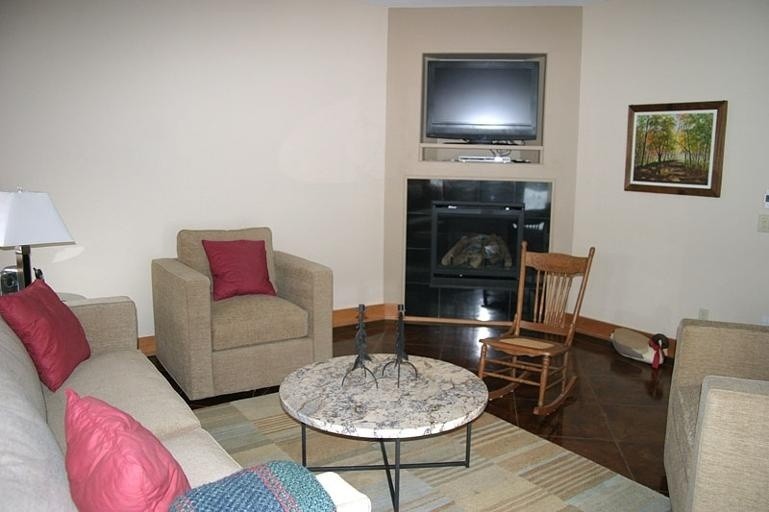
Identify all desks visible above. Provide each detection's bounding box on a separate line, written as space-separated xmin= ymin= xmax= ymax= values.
xmin=281 ymin=353 xmax=488 ymax=512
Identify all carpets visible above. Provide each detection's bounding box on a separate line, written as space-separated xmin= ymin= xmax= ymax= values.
xmin=191 ymin=392 xmax=670 ymax=512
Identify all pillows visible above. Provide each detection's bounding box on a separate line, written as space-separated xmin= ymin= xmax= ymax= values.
xmin=64 ymin=388 xmax=188 ymax=512
xmin=201 ymin=239 xmax=275 ymax=300
xmin=0 ymin=275 xmax=91 ymax=391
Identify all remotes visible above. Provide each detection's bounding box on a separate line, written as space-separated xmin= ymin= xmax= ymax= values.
xmin=512 ymin=159 xmax=530 ymax=163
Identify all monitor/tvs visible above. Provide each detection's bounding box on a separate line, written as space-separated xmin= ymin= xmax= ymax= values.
xmin=425 ymin=60 xmax=540 ymax=145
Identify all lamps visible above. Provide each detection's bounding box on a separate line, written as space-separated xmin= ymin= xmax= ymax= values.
xmin=0 ymin=190 xmax=75 ymax=286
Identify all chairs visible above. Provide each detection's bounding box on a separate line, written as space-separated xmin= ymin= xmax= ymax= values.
xmin=663 ymin=317 xmax=769 ymax=512
xmin=479 ymin=242 xmax=595 ymax=417
xmin=150 ymin=227 xmax=335 ymax=400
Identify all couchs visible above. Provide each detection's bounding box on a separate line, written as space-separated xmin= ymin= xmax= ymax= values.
xmin=0 ymin=297 xmax=370 ymax=512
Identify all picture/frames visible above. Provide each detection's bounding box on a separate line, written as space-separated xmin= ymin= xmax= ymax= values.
xmin=622 ymin=100 xmax=728 ymax=197
